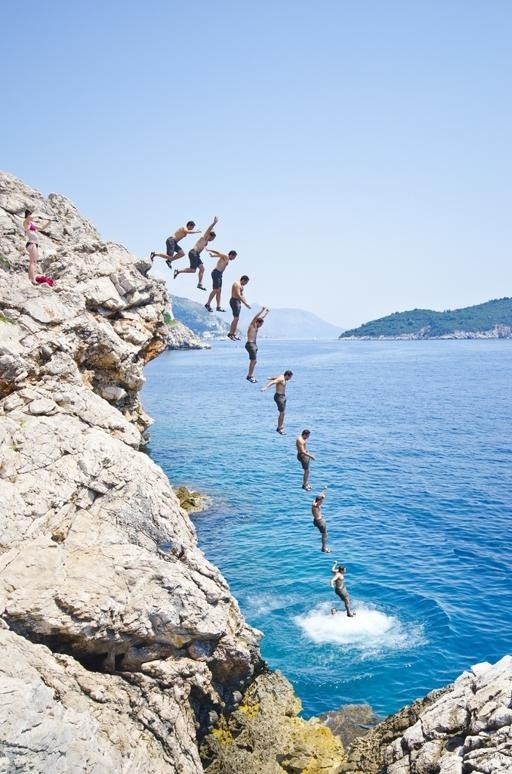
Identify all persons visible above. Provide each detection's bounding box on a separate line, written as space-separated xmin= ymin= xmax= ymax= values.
xmin=330 ymin=607 xmax=337 ymax=615
xmin=261 ymin=370 xmax=294 ymax=435
xmin=245 ymin=306 xmax=270 ymax=383
xmin=23 ymin=207 xmax=53 ymax=284
xmin=203 ymin=247 xmax=238 ymax=311
xmin=150 ymin=220 xmax=203 ymax=267
xmin=173 ymin=216 xmax=220 ymax=291
xmin=296 ymin=430 xmax=316 ymax=490
xmin=226 ymin=274 xmax=252 ymax=341
xmin=311 ymin=484 xmax=331 ymax=552
xmin=329 ymin=560 xmax=356 ymax=617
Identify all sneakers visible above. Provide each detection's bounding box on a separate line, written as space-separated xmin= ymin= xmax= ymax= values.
xmin=228 ymin=333 xmax=240 ymax=342
xmin=166 ymin=259 xmax=171 ymax=268
xmin=303 ymin=482 xmax=311 ymax=491
xmin=150 ymin=251 xmax=155 ymax=262
xmin=217 ymin=307 xmax=224 ymax=312
xmin=174 ymin=269 xmax=178 ymax=279
xmin=348 ymin=611 xmax=357 ymax=617
xmin=277 ymin=427 xmax=286 ymax=436
xmin=197 ymin=283 xmax=206 ymax=290
xmin=247 ymin=375 xmax=257 ymax=383
xmin=322 ymin=547 xmax=330 ymax=552
xmin=205 ymin=305 xmax=212 ymax=312
